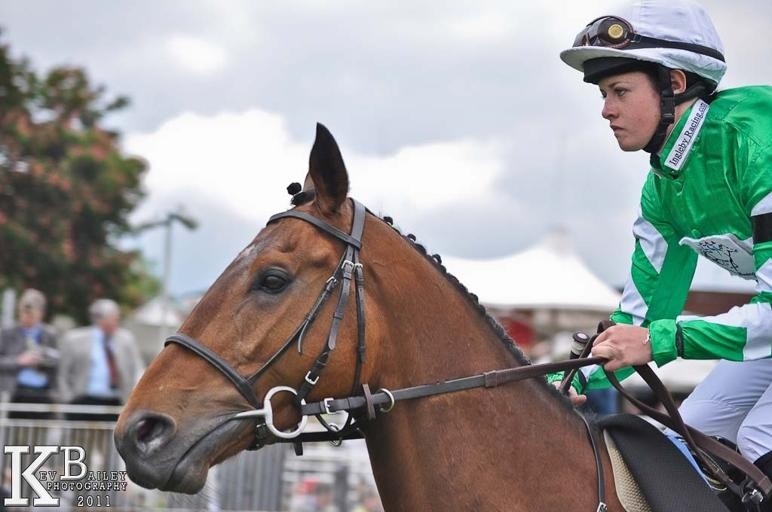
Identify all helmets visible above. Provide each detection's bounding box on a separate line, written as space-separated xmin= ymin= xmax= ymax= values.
xmin=560 ymin=0 xmax=727 ymax=97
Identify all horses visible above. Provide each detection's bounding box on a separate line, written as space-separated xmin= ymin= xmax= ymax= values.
xmin=111 ymin=122 xmax=761 ymax=512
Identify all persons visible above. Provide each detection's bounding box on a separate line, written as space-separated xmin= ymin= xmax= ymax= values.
xmin=0 ymin=288 xmax=59 ymax=504
xmin=59 ymin=297 xmax=144 ymax=508
xmin=493 ymin=307 xmax=537 ymax=362
xmin=547 ymin=0 xmax=772 ymax=512
